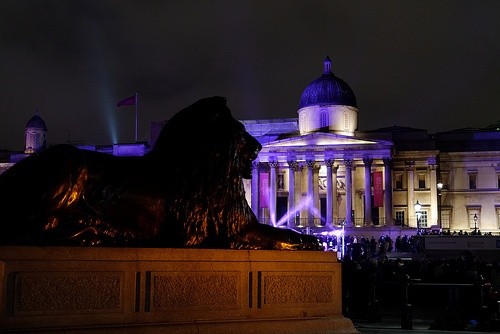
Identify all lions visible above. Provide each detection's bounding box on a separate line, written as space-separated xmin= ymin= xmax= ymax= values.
xmin=1 ymin=96 xmax=325 ymax=252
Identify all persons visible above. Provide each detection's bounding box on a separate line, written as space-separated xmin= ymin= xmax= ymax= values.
xmin=307 ymin=223 xmax=500 ymax=274
